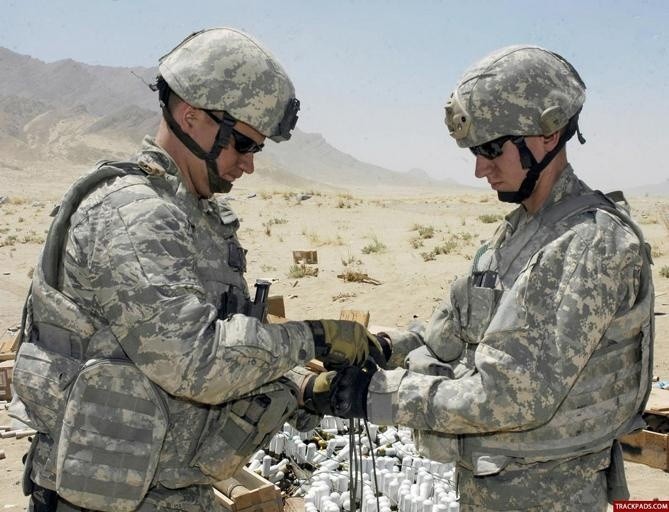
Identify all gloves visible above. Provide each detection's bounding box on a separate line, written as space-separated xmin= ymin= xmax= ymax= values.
xmin=302 ymin=318 xmax=391 ymax=422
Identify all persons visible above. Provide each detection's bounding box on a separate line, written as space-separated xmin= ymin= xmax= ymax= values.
xmin=12 ymin=26 xmax=391 ymax=512
xmin=290 ymin=44 xmax=656 ymax=512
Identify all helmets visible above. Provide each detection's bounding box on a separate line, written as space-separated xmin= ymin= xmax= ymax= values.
xmin=443 ymin=44 xmax=587 ymax=149
xmin=155 ymin=25 xmax=301 ymax=144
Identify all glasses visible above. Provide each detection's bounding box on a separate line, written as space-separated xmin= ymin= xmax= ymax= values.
xmin=202 ymin=108 xmax=265 ymax=155
xmin=468 ymin=134 xmax=523 ymax=160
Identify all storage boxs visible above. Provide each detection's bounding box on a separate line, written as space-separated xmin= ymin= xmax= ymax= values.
xmin=212 ymin=466 xmax=283 ymax=512
xmin=619 ymin=388 xmax=669 ymax=471
